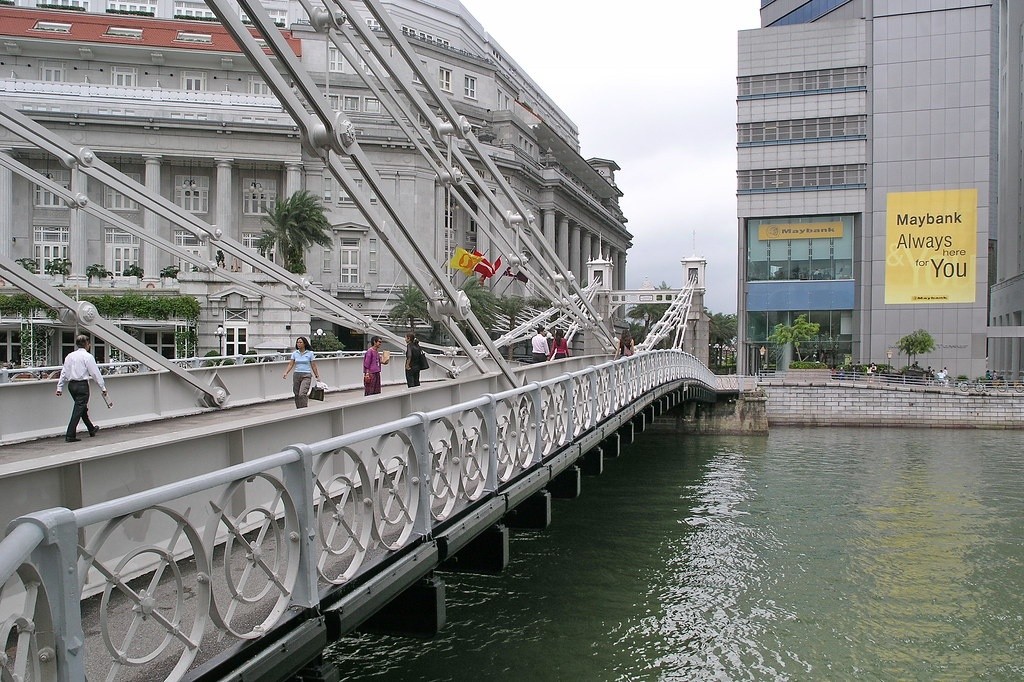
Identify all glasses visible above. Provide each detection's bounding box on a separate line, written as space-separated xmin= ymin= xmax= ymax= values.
xmin=544 ymin=331 xmax=546 ymax=332
xmin=87 ymin=343 xmax=89 ymax=345
xmin=377 ymin=341 xmax=381 ymax=343
xmin=297 ymin=342 xmax=304 ymax=344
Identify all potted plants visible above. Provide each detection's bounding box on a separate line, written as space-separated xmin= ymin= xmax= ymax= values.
xmin=86 ymin=264 xmax=114 ymax=283
xmin=45 ymin=258 xmax=72 ymax=283
xmin=159 ymin=265 xmax=181 ymax=285
xmin=123 ymin=264 xmax=145 ymax=285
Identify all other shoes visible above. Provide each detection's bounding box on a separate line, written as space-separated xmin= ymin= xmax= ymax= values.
xmin=89 ymin=425 xmax=99 ymax=436
xmin=65 ymin=436 xmax=81 ymax=442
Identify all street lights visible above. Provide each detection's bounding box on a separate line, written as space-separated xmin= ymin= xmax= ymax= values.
xmin=713 ymin=343 xmax=721 ymax=369
xmin=312 ymin=328 xmax=327 ymax=351
xmin=886 ymin=350 xmax=895 ymax=373
xmin=722 ymin=345 xmax=730 ymax=369
xmin=759 ymin=346 xmax=766 ymax=376
xmin=729 ymin=348 xmax=736 ymax=369
xmin=213 ymin=327 xmax=228 ymax=356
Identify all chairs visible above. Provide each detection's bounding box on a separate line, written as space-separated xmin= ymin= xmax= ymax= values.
xmin=814 ymin=273 xmax=821 ymax=280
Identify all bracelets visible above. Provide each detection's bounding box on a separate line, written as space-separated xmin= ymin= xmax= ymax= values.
xmin=366 ymin=372 xmax=369 ymax=376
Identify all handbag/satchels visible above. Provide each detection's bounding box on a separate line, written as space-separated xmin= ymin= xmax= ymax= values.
xmin=309 ymin=380 xmax=324 ymax=401
xmin=624 ymin=344 xmax=632 ymax=355
xmin=382 ymin=350 xmax=390 ymax=363
xmin=415 ymin=342 xmax=429 ymax=370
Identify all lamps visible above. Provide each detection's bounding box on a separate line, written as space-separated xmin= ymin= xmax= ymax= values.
xmin=250 ymin=162 xmax=263 ymax=193
xmin=40 ymin=153 xmax=54 ymax=182
xmin=182 ymin=160 xmax=197 ymax=192
xmin=11 ymin=237 xmax=16 ymax=248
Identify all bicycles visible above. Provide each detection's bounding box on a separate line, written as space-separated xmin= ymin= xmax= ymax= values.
xmin=1014 ymin=381 xmax=1024 ymax=392
xmin=983 ymin=378 xmax=1008 ymax=393
xmin=960 ymin=380 xmax=984 ymax=392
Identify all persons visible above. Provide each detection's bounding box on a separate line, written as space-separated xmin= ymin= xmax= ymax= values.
xmin=531 ymin=325 xmax=550 ymax=363
xmin=936 ymin=367 xmax=951 ymax=388
xmin=839 ymin=367 xmax=845 ymax=380
xmin=613 ymin=329 xmax=635 ymax=361
xmin=925 ymin=365 xmax=935 ymax=387
xmin=8 ymin=360 xmax=14 ymax=382
xmin=55 ymin=335 xmax=108 ymax=442
xmin=282 ymin=336 xmax=320 ymax=409
xmin=363 ymin=335 xmax=389 ymax=396
xmin=549 ymin=328 xmax=570 ymax=361
xmin=108 ymin=355 xmax=115 ymax=374
xmin=867 ymin=362 xmax=877 ymax=383
xmin=404 ymin=333 xmax=421 ymax=388
xmin=830 ymin=367 xmax=836 ymax=379
xmin=40 ymin=356 xmax=46 ymax=379
xmin=993 ymin=370 xmax=998 ymax=380
xmin=985 ymin=370 xmax=992 ymax=380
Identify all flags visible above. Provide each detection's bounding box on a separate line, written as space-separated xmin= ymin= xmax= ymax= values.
xmin=502 ymin=266 xmax=514 ymax=276
xmin=448 ymin=245 xmax=482 ymax=277
xmin=471 ymin=249 xmax=502 ymax=278
xmin=515 ymin=270 xmax=529 ymax=283
